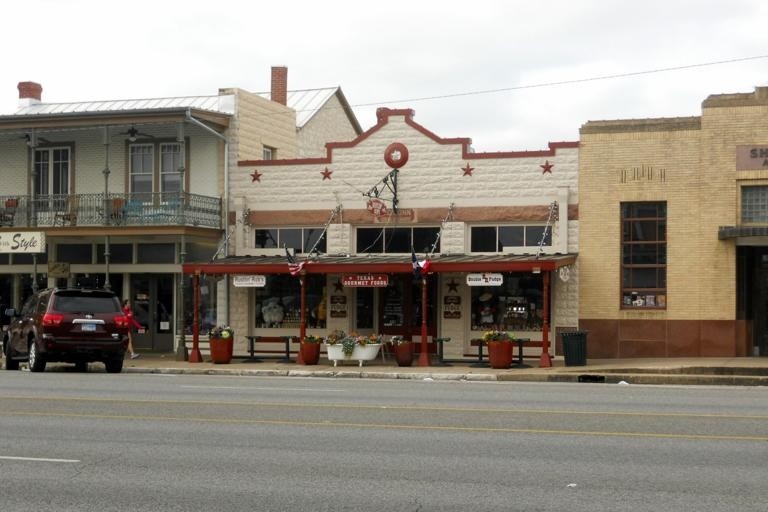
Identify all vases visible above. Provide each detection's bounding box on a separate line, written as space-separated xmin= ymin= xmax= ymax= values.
xmin=210 ymin=337 xmax=233 ymax=363
xmin=395 ymin=342 xmax=415 ymax=365
xmin=301 ymin=343 xmax=320 ymax=363
xmin=488 ymin=341 xmax=514 ymax=369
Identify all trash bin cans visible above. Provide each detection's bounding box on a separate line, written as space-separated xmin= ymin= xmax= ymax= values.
xmin=560 ymin=331 xmax=588 ymax=367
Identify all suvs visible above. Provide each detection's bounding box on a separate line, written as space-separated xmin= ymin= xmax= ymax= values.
xmin=1 ymin=286 xmax=131 ymax=378
xmin=134 ymin=293 xmax=171 ymax=333
xmin=502 ymin=301 xmax=538 ymax=329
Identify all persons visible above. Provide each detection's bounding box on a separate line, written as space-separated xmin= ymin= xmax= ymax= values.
xmin=122 ymin=298 xmax=146 ymax=361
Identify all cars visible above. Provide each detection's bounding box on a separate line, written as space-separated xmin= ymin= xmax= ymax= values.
xmin=199 ymin=308 xmax=216 ymax=331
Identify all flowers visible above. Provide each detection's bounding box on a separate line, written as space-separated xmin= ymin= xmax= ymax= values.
xmin=322 ymin=331 xmax=382 ymax=357
xmin=301 ymin=336 xmax=321 ymax=344
xmin=210 ymin=324 xmax=233 ymax=339
xmin=481 ymin=328 xmax=517 ymax=344
xmin=390 ymin=334 xmax=403 ymax=346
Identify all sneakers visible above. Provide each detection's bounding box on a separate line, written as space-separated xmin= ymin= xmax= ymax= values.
xmin=130 ymin=353 xmax=141 ymax=359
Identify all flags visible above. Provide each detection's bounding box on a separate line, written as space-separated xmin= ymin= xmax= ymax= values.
xmin=417 ymin=254 xmax=431 ymax=274
xmin=411 ymin=252 xmax=423 ymax=277
xmin=284 ymin=247 xmax=309 ymax=277
